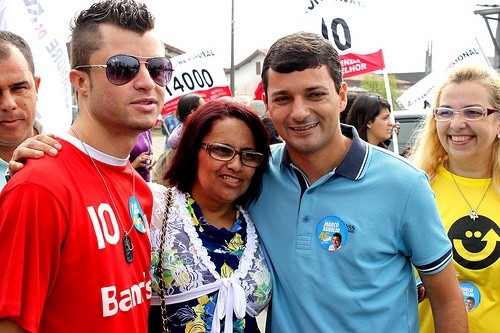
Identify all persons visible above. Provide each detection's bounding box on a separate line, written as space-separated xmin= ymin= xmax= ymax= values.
xmin=0 ymin=30 xmax=63 ymax=196
xmin=161 ymin=93 xmax=207 ymax=150
xmin=339 ymin=87 xmax=400 ymax=152
xmin=5 ymin=96 xmax=429 ymax=333
xmin=1 ymin=0 xmax=166 ymax=333
xmin=128 ymin=127 xmax=161 ymax=182
xmin=241 ymin=31 xmax=470 ymax=333
xmin=405 ymin=64 xmax=500 ymax=333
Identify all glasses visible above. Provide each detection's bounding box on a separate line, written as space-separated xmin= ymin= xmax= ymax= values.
xmin=75 ymin=55 xmax=174 ymax=87
xmin=433 ymin=107 xmax=500 ymax=122
xmin=201 ymin=143 xmax=264 ymax=168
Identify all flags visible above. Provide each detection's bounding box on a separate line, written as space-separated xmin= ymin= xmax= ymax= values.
xmin=396 ymin=29 xmax=496 ymax=114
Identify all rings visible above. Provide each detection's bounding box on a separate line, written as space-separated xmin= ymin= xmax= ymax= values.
xmin=140 ymin=156 xmax=142 ymax=160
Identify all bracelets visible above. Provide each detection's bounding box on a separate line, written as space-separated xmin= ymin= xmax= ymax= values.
xmin=4 ymin=165 xmax=11 ymax=182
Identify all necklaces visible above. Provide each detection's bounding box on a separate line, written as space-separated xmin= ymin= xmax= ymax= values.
xmin=70 ymin=125 xmax=137 ymax=264
xmin=446 ymin=158 xmax=493 ymax=220
